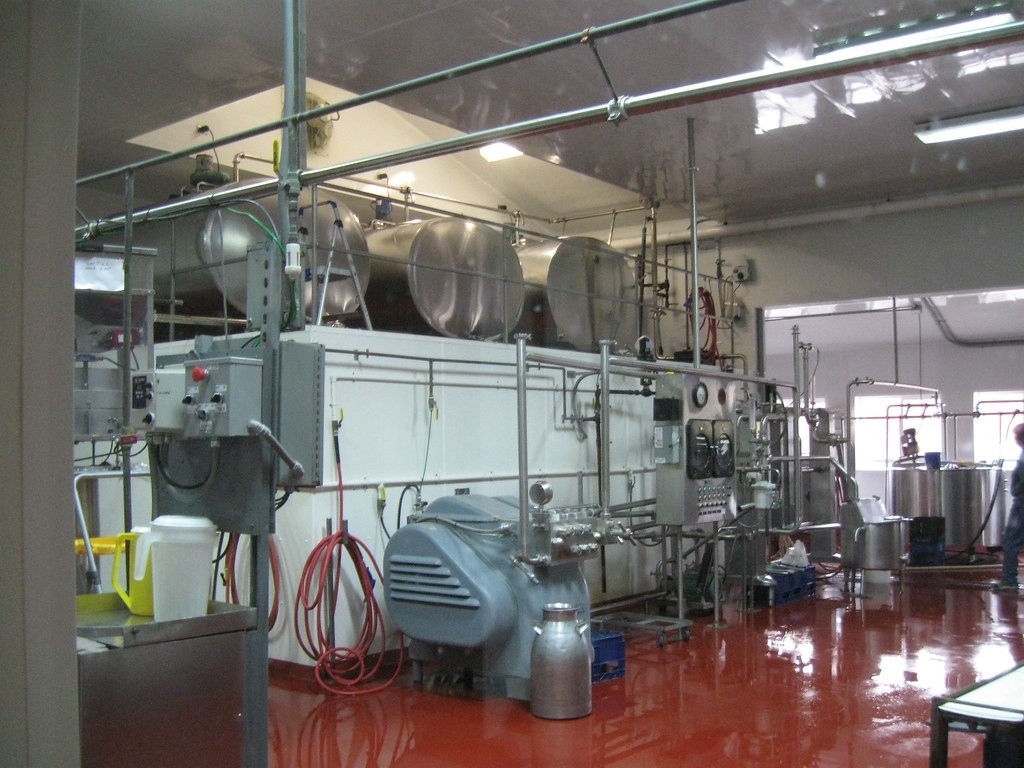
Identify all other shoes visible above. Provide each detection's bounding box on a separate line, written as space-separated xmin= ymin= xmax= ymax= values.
xmin=990 ymin=579 xmax=1018 ymax=593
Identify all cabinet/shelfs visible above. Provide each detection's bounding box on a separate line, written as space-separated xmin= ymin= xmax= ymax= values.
xmin=77 ymin=591 xmax=268 ymax=767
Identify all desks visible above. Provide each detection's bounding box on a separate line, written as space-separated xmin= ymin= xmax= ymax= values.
xmin=930 ymin=660 xmax=1024 ymax=768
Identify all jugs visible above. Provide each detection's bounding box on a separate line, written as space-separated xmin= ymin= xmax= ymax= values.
xmin=112 ymin=527 xmax=155 ymax=617
xmin=134 ymin=515 xmax=218 ymax=623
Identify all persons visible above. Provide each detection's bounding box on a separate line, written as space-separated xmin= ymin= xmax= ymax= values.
xmin=985 ymin=422 xmax=1024 ymax=595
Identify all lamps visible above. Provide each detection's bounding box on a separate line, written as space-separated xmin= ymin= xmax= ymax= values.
xmin=913 ymin=105 xmax=1024 ymax=144
xmin=813 ymin=5 xmax=1018 ymax=63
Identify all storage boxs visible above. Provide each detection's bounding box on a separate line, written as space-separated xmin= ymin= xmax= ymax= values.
xmin=771 ymin=564 xmax=818 ymax=604
xmin=588 ymin=629 xmax=626 ymax=681
